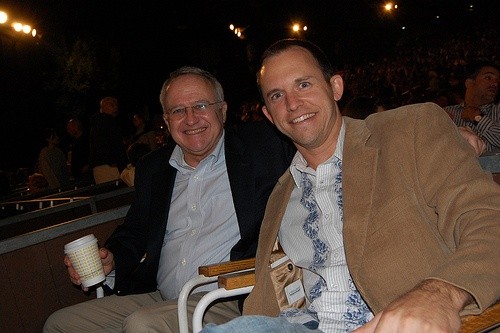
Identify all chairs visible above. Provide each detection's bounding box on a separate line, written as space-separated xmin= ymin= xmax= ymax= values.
xmin=97 ymin=145 xmax=500 ymax=333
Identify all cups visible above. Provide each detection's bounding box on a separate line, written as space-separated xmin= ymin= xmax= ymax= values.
xmin=63 ymin=234 xmax=106 ymax=288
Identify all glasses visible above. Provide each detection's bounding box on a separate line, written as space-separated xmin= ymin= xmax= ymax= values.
xmin=165 ymin=101 xmax=220 ymax=120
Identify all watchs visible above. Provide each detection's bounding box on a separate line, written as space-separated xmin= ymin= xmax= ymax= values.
xmin=473 ymin=114 xmax=484 ymax=124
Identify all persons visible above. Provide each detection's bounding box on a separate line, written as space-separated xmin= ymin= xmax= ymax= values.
xmin=330 ymin=33 xmax=500 ymax=154
xmin=235 ymin=98 xmax=265 ymax=122
xmin=17 ymin=97 xmax=170 ymax=197
xmin=199 ymin=39 xmax=500 ymax=333
xmin=44 ymin=65 xmax=286 ymax=333
